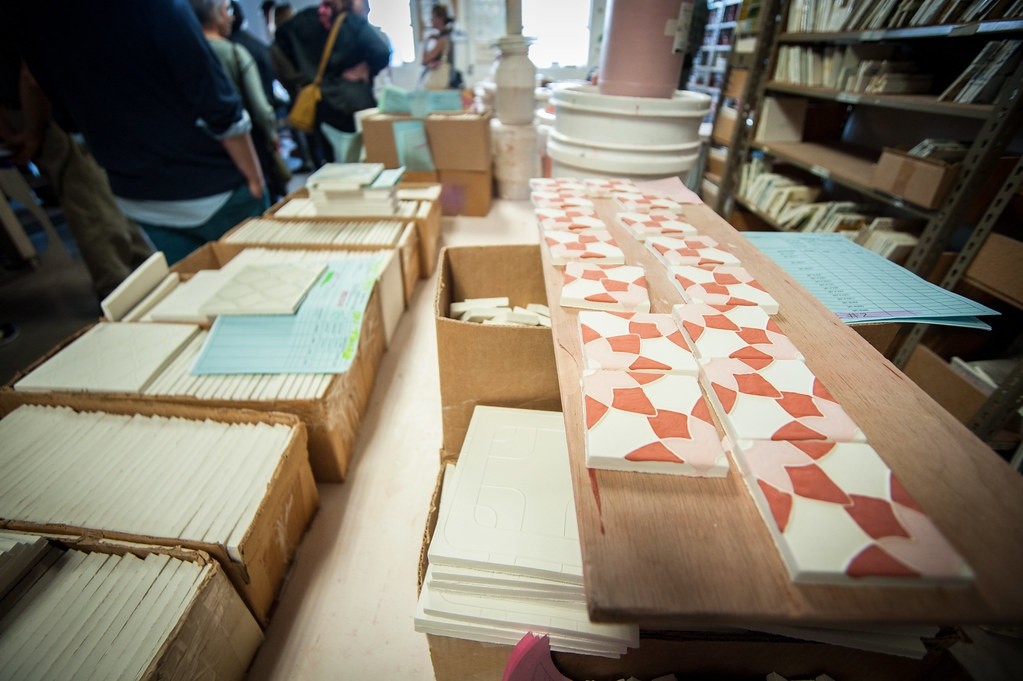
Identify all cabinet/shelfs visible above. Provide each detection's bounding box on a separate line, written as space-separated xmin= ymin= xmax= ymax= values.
xmin=674 ymin=1 xmax=1022 ymax=482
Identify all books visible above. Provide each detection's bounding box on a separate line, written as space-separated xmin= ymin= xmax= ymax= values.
xmin=729 ymin=0 xmax=1023 ymax=479
xmin=2 ymin=164 xmax=1023 ymax=680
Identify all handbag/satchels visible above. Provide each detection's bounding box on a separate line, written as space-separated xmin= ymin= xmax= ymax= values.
xmin=285 ymin=85 xmax=320 ymax=130
xmin=248 ymin=122 xmax=291 ymax=198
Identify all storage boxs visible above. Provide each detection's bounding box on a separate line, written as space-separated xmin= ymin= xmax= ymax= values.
xmin=2 ymin=77 xmax=893 ymax=681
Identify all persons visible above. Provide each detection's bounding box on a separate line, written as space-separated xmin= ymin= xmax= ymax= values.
xmin=417 ymin=2 xmax=456 ymax=90
xmin=259 ymin=0 xmax=392 ymax=175
xmin=14 ymin=0 xmax=272 ymax=265
xmin=188 ymin=0 xmax=285 ymax=211
xmin=224 ymin=0 xmax=279 ymax=119
